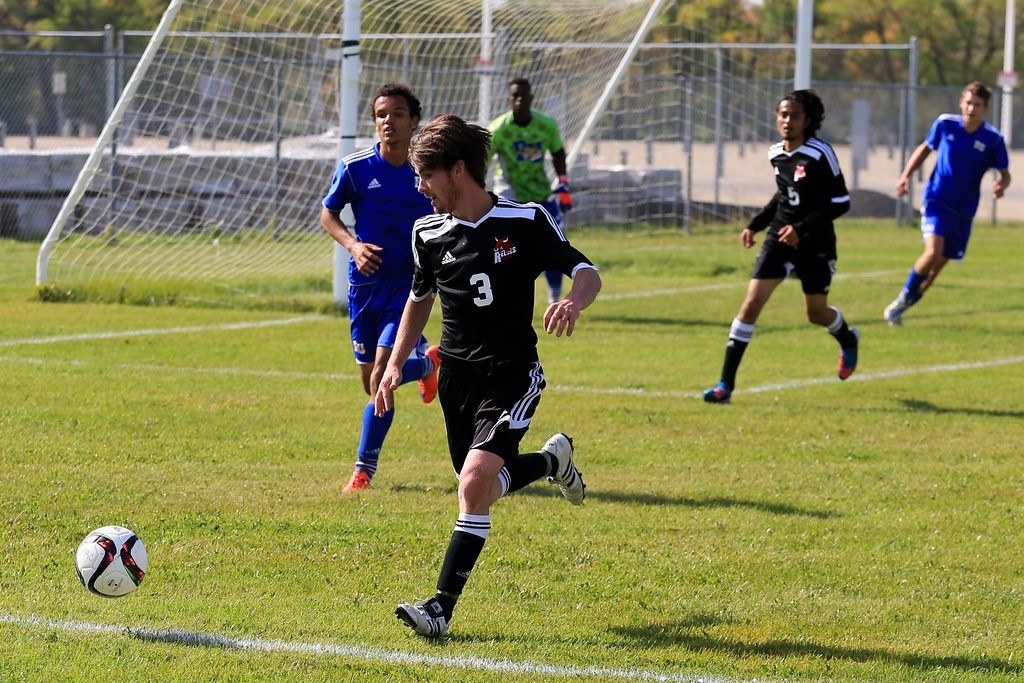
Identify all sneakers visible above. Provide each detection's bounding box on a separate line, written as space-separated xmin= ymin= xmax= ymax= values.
xmin=395 ymin=598 xmax=450 ymax=639
xmin=418 ymin=345 xmax=441 ymax=404
xmin=343 ymin=472 xmax=372 ymax=492
xmin=838 ymin=328 xmax=862 ymax=380
xmin=703 ymin=383 xmax=731 ymax=403
xmin=542 ymin=433 xmax=586 ymax=506
xmin=885 ymin=291 xmax=911 ymax=326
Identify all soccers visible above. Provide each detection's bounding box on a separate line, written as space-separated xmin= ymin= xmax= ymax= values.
xmin=74 ymin=525 xmax=149 ymax=599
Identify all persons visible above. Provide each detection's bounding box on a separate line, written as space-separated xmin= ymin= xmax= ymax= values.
xmin=883 ymin=80 xmax=1012 ymax=325
xmin=320 ymin=84 xmax=442 ymax=494
xmin=375 ymin=114 xmax=602 ymax=639
xmin=486 ymin=78 xmax=572 ymax=304
xmin=704 ymin=90 xmax=859 ymax=404
